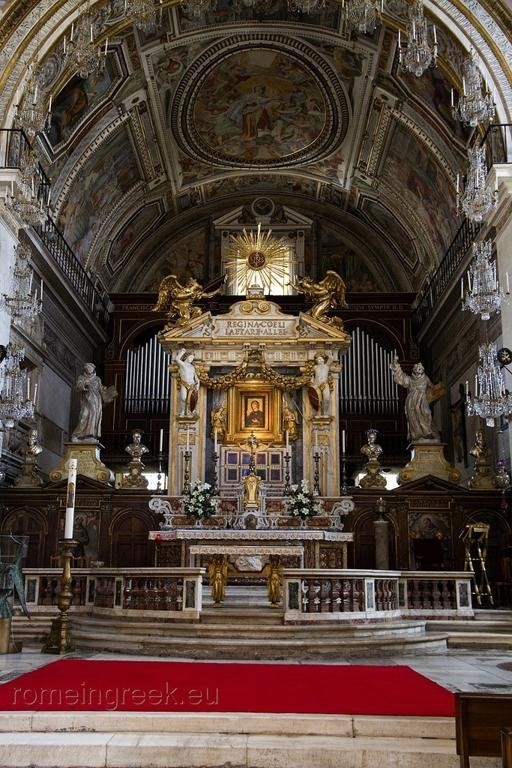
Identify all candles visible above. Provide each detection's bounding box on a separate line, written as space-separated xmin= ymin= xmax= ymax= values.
xmin=342 ymin=429 xmax=347 ymax=452
xmin=314 ymin=429 xmax=318 ymax=452
xmin=285 ymin=430 xmax=289 ymax=453
xmin=160 ymin=428 xmax=163 ymax=451
xmin=187 ymin=430 xmax=190 ymax=452
xmin=63 ymin=457 xmax=78 ymax=541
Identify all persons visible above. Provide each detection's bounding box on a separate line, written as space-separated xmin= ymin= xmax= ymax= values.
xmin=308 ymin=349 xmax=333 ymax=419
xmin=414 ymin=517 xmax=444 ymax=540
xmin=357 ymin=356 xmax=447 ymax=461
xmin=176 ymin=348 xmax=200 ymax=420
xmin=70 ymin=363 xmax=157 ymax=463
xmin=286 ymin=276 xmax=337 ymax=327
xmin=72 ymin=514 xmax=89 ymax=559
xmin=245 ymin=399 xmax=264 ymax=426
xmin=169 ymin=277 xmax=220 ymax=323
xmin=207 ymin=555 xmax=230 ymax=607
xmin=260 ymin=554 xmax=285 ymax=608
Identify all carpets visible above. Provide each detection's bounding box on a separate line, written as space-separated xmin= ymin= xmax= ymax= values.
xmin=0 ymin=659 xmax=458 ymax=718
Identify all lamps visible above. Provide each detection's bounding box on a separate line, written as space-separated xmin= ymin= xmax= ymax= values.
xmin=342 ymin=0 xmax=439 ymax=77
xmin=62 ymin=0 xmax=165 ymax=81
xmin=448 ymin=0 xmax=512 ymax=431
xmin=1 ymin=2 xmax=54 ymax=430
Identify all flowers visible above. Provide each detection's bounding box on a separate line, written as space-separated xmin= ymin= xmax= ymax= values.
xmin=181 ymin=477 xmax=223 ymax=521
xmin=287 ymin=477 xmax=325 ymax=518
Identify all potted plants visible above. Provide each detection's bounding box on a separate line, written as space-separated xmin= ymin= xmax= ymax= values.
xmin=213 ymin=427 xmax=218 ymax=452
